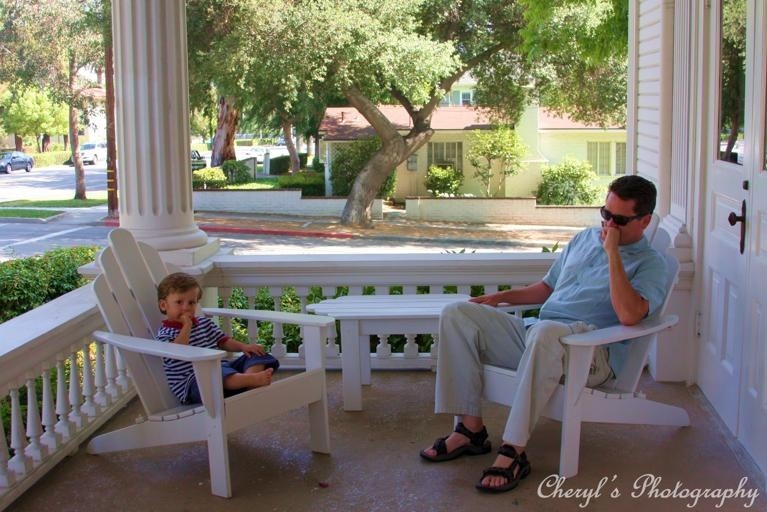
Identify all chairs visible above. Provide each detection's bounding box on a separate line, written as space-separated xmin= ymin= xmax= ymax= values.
xmin=86 ymin=229 xmax=337 ymax=499
xmin=452 ymin=213 xmax=689 ymax=479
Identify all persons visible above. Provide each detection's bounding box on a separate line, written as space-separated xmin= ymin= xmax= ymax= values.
xmin=155 ymin=271 xmax=279 ymax=405
xmin=421 ymin=172 xmax=672 ymax=493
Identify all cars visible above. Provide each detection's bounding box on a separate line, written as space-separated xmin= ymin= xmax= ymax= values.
xmin=190 ymin=150 xmax=206 ymax=170
xmin=277 ymin=139 xmax=293 ymax=146
xmin=0 ymin=152 xmax=35 ymax=174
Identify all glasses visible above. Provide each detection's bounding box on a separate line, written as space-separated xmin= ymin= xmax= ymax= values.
xmin=600 ymin=206 xmax=638 ymax=226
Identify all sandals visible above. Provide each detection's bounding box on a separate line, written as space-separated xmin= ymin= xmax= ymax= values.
xmin=420 ymin=423 xmax=492 ymax=461
xmin=476 ymin=445 xmax=531 ymax=493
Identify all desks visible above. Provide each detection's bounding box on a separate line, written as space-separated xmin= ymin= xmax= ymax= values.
xmin=305 ymin=294 xmax=476 ymax=411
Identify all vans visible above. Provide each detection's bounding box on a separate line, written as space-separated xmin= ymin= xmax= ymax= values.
xmin=71 ymin=143 xmax=107 ymax=166
